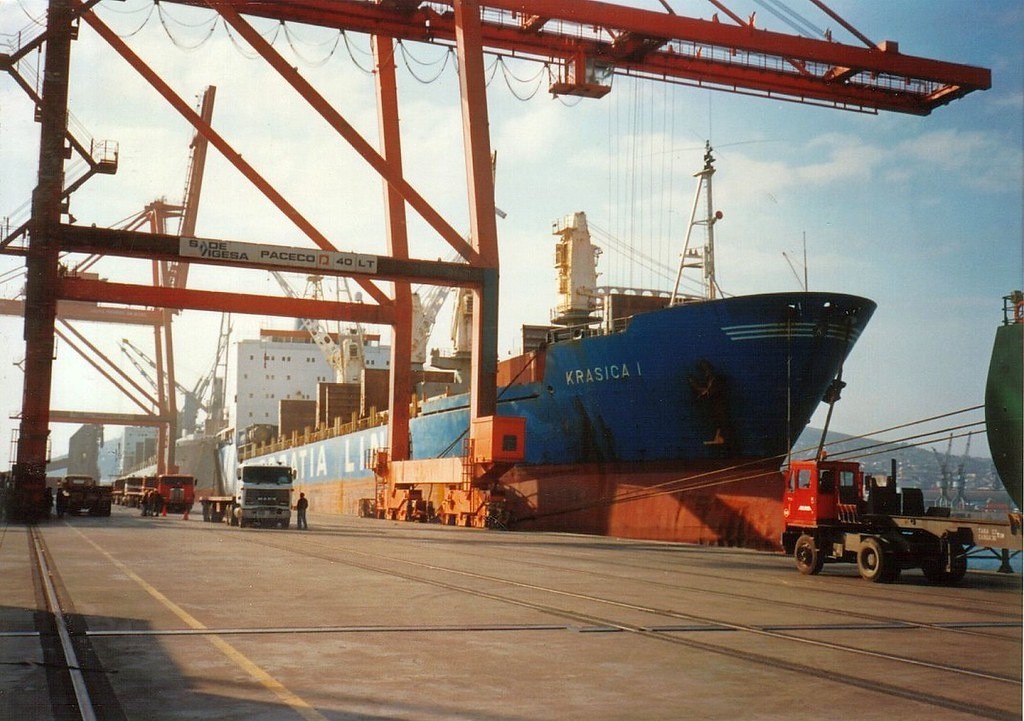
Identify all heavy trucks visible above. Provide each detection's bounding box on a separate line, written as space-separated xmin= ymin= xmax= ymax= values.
xmin=200 ymin=464 xmax=300 ymax=528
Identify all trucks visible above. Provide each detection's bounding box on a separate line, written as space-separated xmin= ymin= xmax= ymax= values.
xmin=780 ymin=450 xmax=976 ymax=582
xmin=55 ymin=471 xmax=198 ymax=517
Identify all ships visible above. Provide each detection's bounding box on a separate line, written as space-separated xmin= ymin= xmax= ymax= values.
xmin=229 ymin=140 xmax=880 ymax=548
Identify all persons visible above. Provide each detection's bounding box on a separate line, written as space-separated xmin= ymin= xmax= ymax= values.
xmin=148 ymin=491 xmax=154 ymax=516
xmin=295 ymin=493 xmax=308 ymax=529
xmin=138 ymin=490 xmax=148 ymax=515
xmin=153 ymin=491 xmax=161 ymax=516
